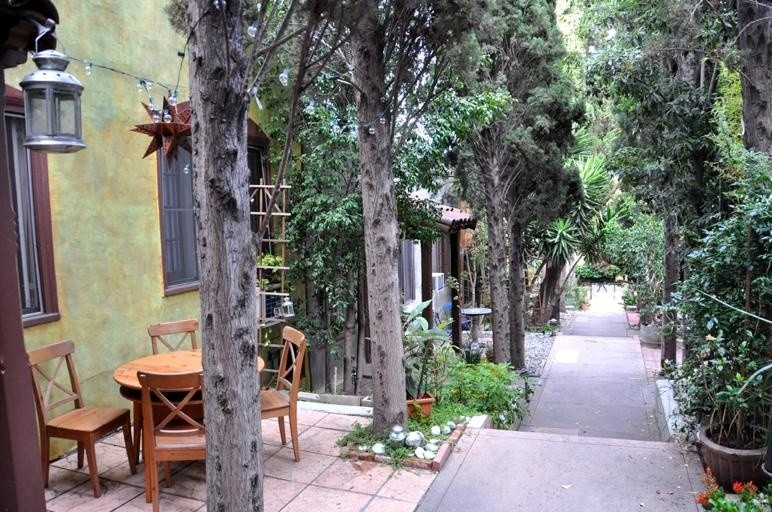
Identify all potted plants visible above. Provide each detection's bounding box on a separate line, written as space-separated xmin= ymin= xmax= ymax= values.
xmin=400 ymin=307 xmax=450 ymax=423
xmin=657 ymin=198 xmax=772 ymax=495
xmin=579 ymin=286 xmax=591 ymax=311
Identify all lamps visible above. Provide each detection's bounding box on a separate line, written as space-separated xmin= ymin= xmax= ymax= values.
xmin=15 ymin=24 xmax=91 ymax=163
